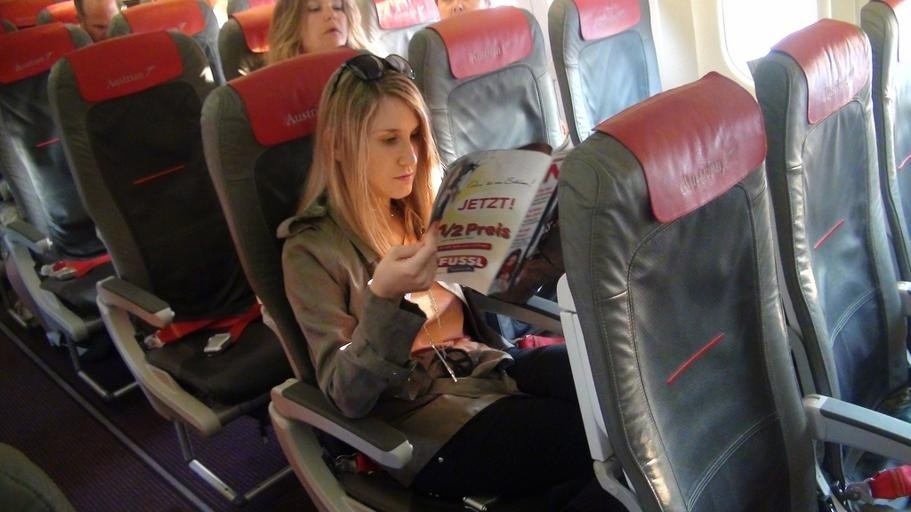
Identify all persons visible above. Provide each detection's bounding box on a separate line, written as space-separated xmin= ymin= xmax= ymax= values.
xmin=266 ymin=1 xmax=368 ymax=66
xmin=432 ymin=0 xmax=498 ymax=21
xmin=282 ymin=51 xmax=622 ymax=510
xmin=72 ymin=1 xmax=123 ymax=44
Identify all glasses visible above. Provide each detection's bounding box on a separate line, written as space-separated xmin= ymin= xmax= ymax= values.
xmin=329 ymin=53 xmax=417 ymax=99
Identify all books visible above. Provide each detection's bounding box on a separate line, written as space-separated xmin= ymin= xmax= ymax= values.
xmin=420 ymin=129 xmax=570 ymax=296
